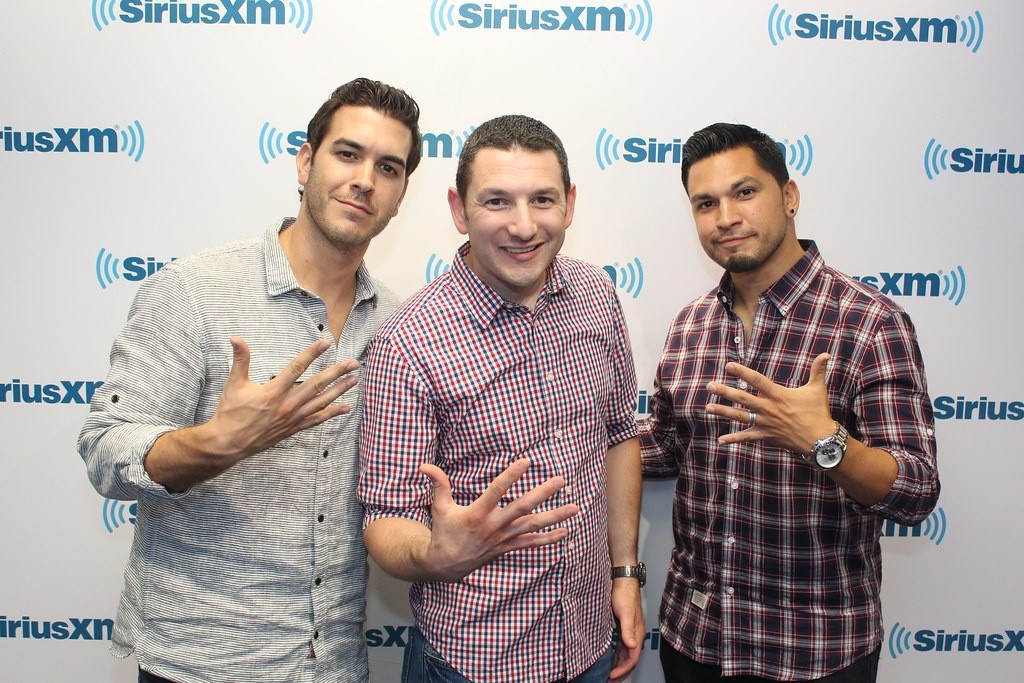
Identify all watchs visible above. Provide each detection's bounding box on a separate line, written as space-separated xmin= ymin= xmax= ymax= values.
xmin=801 ymin=421 xmax=849 ymax=473
xmin=610 ymin=562 xmax=646 ymax=588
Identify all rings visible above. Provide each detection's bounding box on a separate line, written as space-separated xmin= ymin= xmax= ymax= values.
xmin=748 ymin=409 xmax=756 ymax=424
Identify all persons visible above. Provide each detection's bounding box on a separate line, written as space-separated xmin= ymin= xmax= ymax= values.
xmin=78 ymin=74 xmax=422 ymax=683
xmin=633 ymin=124 xmax=940 ymax=683
xmin=356 ymin=118 xmax=646 ymax=683
